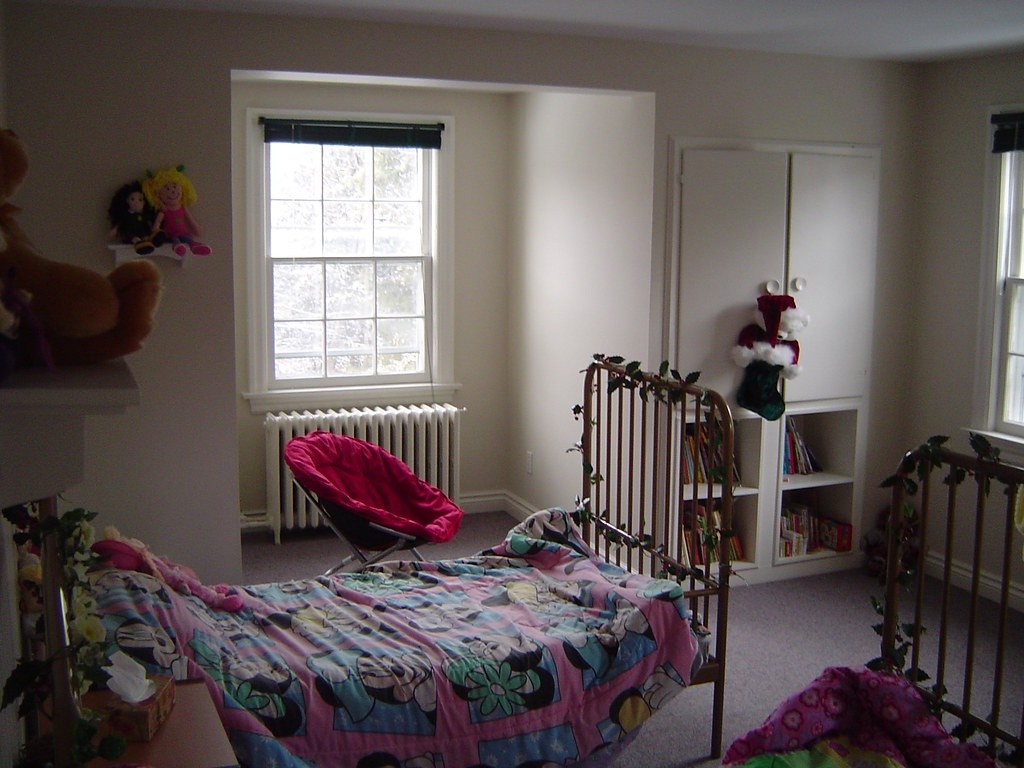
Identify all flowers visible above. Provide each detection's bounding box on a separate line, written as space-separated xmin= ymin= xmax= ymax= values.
xmin=0 ymin=492 xmax=125 ymax=768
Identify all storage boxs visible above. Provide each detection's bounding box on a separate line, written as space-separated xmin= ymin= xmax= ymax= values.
xmin=819 ymin=515 xmax=852 ymax=552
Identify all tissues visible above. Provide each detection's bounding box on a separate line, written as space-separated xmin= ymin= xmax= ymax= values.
xmin=101 ymin=650 xmax=176 ymax=744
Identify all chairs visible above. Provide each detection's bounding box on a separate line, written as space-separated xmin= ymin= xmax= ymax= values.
xmin=284 ymin=431 xmax=465 ymax=576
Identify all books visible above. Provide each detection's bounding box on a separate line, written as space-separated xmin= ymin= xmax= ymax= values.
xmin=680 ymin=420 xmax=744 ymax=568
xmin=778 ymin=415 xmax=819 ymax=559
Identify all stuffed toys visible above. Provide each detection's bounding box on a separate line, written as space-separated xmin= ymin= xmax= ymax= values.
xmin=731 ymin=294 xmax=799 ymax=420
xmin=0 ymin=128 xmax=162 ymax=368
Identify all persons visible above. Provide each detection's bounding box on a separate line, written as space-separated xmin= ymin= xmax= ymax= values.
xmin=106 ymin=180 xmax=166 ymax=255
xmin=142 ymin=164 xmax=213 ymax=257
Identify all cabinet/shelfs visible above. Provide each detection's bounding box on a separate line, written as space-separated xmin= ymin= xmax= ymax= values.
xmin=668 ymin=136 xmax=882 ymax=590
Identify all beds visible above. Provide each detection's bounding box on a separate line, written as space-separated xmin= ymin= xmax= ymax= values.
xmin=682 ymin=431 xmax=1024 ymax=768
xmin=15 ymin=355 xmax=734 ymax=768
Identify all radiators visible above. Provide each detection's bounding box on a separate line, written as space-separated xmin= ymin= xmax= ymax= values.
xmin=262 ymin=403 xmax=462 ymax=545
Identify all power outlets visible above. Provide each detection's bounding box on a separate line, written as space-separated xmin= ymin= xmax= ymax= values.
xmin=526 ymin=451 xmax=532 ymax=475
